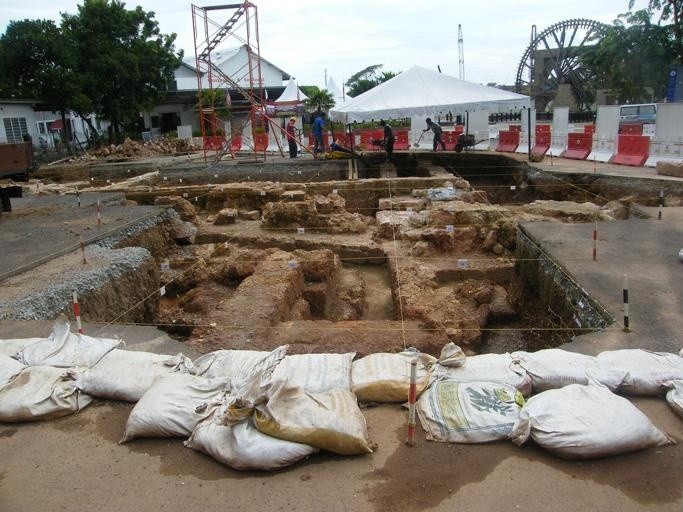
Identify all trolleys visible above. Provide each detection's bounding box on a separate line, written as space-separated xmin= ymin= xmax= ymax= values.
xmin=455 ymin=134 xmax=495 ymax=152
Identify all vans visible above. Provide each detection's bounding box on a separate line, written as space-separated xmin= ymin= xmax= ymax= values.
xmin=620 ymin=103 xmax=657 ymax=123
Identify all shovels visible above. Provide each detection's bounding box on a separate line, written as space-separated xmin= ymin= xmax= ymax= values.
xmin=413 ymin=130 xmax=425 ymax=148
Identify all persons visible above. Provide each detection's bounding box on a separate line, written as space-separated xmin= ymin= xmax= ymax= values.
xmin=311 ymin=112 xmax=329 ymax=159
xmin=379 ymin=120 xmax=395 ymax=162
xmin=287 ymin=117 xmax=299 ymax=159
xmin=422 ymin=118 xmax=446 ymax=151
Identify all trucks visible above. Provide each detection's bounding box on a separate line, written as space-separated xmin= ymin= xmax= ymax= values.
xmin=0 ymin=141 xmax=33 ymax=213
